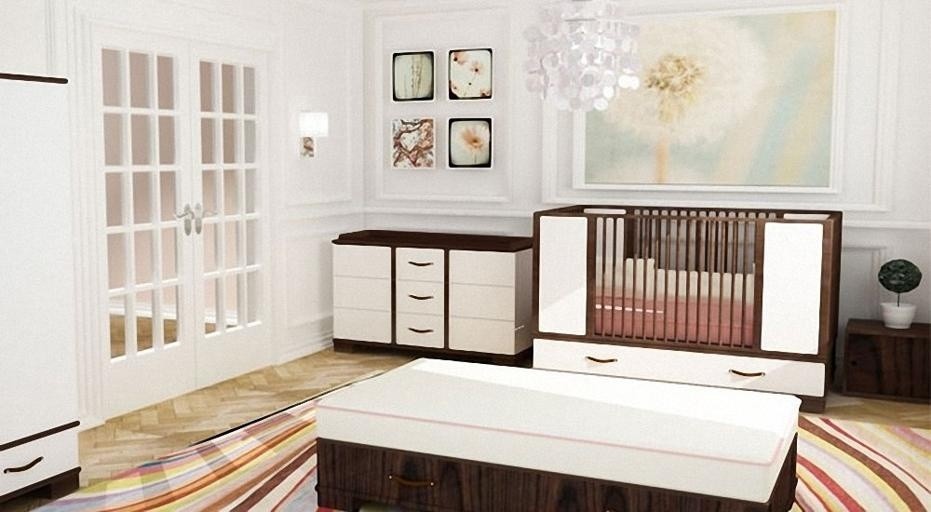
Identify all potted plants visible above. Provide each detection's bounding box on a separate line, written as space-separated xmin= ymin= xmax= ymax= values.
xmin=878 ymin=259 xmax=923 ymax=330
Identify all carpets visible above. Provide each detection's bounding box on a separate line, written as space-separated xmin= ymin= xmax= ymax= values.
xmin=30 ymin=372 xmax=931 ymax=512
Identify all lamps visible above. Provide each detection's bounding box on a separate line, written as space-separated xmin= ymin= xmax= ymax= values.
xmin=299 ymin=112 xmax=330 ymax=157
xmin=525 ymin=0 xmax=638 ymax=112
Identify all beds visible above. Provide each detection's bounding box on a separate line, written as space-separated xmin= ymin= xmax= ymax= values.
xmin=532 ymin=204 xmax=844 ymax=414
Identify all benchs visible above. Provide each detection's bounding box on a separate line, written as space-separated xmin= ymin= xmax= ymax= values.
xmin=318 ymin=354 xmax=799 ymax=512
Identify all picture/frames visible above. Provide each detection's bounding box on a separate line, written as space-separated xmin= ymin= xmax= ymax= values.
xmin=447 ymin=114 xmax=494 ymax=170
xmin=572 ymin=0 xmax=850 ymax=194
xmin=446 ymin=47 xmax=494 ymax=102
xmin=391 ymin=118 xmax=436 ymax=170
xmin=392 ymin=49 xmax=435 ymax=101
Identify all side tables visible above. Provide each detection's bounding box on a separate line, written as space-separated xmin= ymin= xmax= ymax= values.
xmin=844 ymin=318 xmax=931 ymax=404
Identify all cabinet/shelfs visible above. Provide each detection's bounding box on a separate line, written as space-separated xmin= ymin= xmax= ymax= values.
xmin=331 ymin=230 xmax=533 ymax=364
xmin=0 ymin=70 xmax=83 ymax=512
